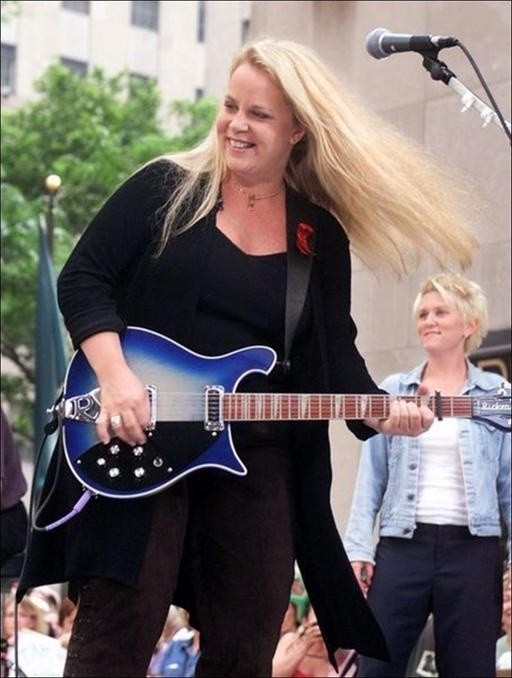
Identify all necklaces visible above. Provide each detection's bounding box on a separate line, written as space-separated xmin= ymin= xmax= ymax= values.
xmin=229 ymin=176 xmax=287 ymax=211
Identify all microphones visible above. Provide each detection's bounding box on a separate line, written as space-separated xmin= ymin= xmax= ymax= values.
xmin=365 ymin=27 xmax=458 ymax=59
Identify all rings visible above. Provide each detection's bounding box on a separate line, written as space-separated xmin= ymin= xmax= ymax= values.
xmin=111 ymin=412 xmax=122 ymax=428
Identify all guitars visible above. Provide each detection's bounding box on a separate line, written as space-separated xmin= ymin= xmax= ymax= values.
xmin=47 ymin=325 xmax=512 ymax=500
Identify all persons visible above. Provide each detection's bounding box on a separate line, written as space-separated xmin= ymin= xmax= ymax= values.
xmin=270 ymin=566 xmax=356 ymax=676
xmin=0 ymin=577 xmax=78 ymax=678
xmin=405 ymin=559 xmax=512 ymax=677
xmin=14 ymin=40 xmax=483 ymax=678
xmin=2 ymin=411 xmax=30 ymax=587
xmin=145 ymin=604 xmax=202 ymax=677
xmin=343 ymin=271 xmax=512 ymax=674
